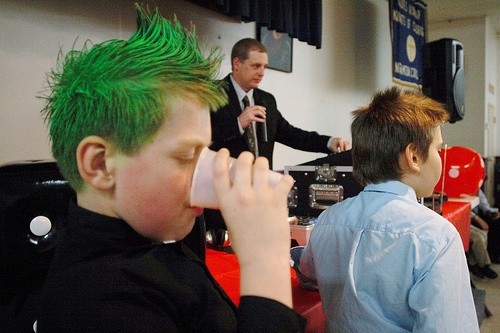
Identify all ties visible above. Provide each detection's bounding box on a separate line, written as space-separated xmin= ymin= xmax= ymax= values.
xmin=242 ymin=94 xmax=256 ymax=159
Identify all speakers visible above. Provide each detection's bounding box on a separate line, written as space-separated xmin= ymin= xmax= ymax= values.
xmin=422 ymin=37 xmax=465 ymax=123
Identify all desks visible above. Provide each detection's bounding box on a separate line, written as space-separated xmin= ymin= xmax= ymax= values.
xmin=205 ymin=201 xmax=471 ymax=333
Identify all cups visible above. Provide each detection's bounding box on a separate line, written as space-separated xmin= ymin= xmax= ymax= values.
xmin=191 ymin=146 xmax=283 ymax=209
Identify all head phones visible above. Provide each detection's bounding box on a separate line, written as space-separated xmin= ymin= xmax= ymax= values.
xmin=205 ymin=229 xmax=234 ymax=254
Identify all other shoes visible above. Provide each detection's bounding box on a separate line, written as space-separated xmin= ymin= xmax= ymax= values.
xmin=481 ymin=264 xmax=497 ymax=278
xmin=468 ymin=263 xmax=484 ymax=278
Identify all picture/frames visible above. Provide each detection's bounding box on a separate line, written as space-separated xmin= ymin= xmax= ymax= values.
xmin=256 ymin=22 xmax=293 ymax=73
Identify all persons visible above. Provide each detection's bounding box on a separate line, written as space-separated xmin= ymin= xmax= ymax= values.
xmin=297 ymin=86 xmax=481 ymax=333
xmin=209 ymin=37 xmax=351 ymax=171
xmin=439 ymin=183 xmax=500 ymax=279
xmin=0 ymin=2 xmax=309 ymax=333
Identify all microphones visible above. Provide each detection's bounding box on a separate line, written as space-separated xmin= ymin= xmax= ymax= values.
xmin=255 ymin=99 xmax=267 ymax=143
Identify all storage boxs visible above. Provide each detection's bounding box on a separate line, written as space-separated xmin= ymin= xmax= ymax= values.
xmin=289 ymin=223 xmax=314 ymax=247
xmin=284 ymin=149 xmax=363 ymax=226
